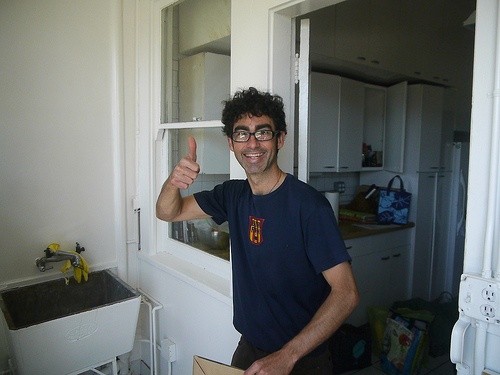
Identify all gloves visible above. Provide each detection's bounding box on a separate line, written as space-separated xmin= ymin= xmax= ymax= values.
xmin=49 ymin=242 xmax=89 ymax=283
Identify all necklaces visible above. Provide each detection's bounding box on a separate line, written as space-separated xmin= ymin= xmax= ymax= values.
xmin=269 ymin=170 xmax=282 ymax=194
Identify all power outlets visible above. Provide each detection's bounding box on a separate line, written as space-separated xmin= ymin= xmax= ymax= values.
xmin=457 ymin=272 xmax=500 ymax=326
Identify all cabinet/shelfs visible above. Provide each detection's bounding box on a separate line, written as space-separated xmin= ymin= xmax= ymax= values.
xmin=176 ymin=0 xmax=476 ymax=325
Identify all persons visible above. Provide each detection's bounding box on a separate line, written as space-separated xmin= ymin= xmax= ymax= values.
xmin=155 ymin=87 xmax=358 ymax=375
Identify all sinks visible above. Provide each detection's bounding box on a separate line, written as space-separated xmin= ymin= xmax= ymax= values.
xmin=0 ymin=267 xmax=141 ymax=375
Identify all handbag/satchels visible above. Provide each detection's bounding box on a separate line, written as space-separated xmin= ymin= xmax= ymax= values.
xmin=377 ymin=175 xmax=412 ymax=225
xmin=329 ymin=291 xmax=458 ymax=375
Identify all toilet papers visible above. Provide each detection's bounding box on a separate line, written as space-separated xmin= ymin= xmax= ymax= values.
xmin=325 ymin=192 xmax=338 ymax=225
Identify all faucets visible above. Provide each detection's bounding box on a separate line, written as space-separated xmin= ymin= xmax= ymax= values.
xmin=34 ymin=244 xmax=85 ymax=272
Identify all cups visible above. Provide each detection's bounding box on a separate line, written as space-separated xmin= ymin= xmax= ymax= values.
xmin=325 ymin=192 xmax=340 ymax=225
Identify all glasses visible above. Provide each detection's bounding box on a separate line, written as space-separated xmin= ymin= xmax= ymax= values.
xmin=227 ymin=128 xmax=280 ymax=142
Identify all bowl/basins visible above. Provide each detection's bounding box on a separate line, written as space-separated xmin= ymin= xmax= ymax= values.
xmin=195 ymin=226 xmax=229 ymax=250
